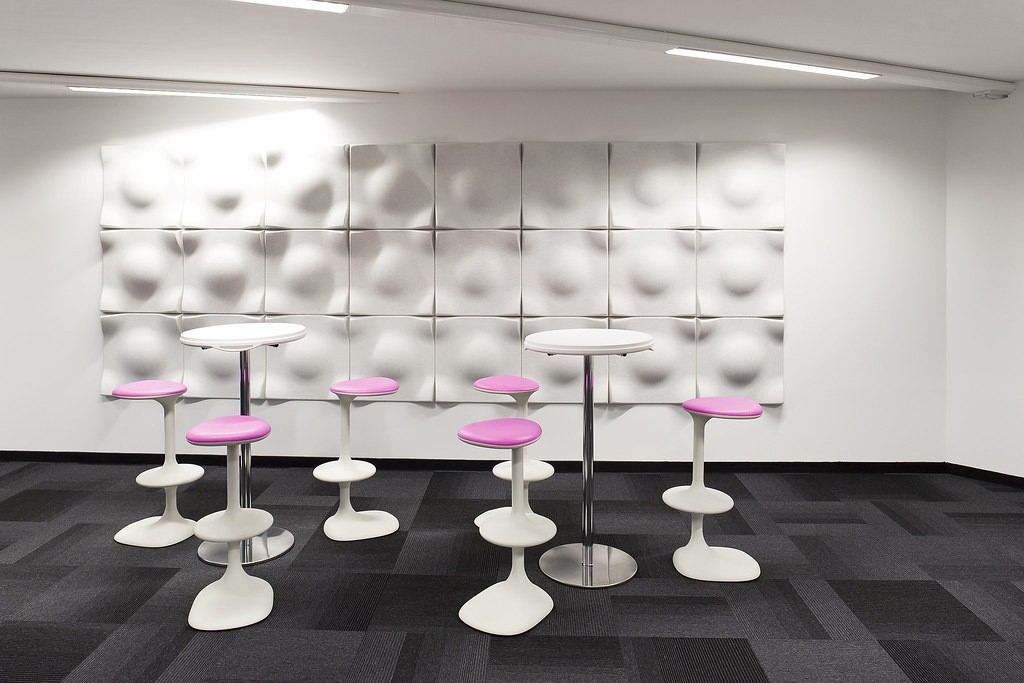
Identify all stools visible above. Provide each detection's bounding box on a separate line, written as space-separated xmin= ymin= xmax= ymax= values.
xmin=185 ymin=415 xmax=277 ymax=632
xmin=456 ymin=417 xmax=557 ymax=636
xmin=315 ymin=377 xmax=402 ymax=542
xmin=663 ymin=394 xmax=764 ymax=584
xmin=472 ymin=375 xmax=556 ymax=528
xmin=114 ymin=381 xmax=205 ymax=548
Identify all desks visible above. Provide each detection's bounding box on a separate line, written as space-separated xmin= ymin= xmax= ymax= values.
xmin=521 ymin=327 xmax=657 ymax=589
xmin=181 ymin=322 xmax=307 ymax=565
